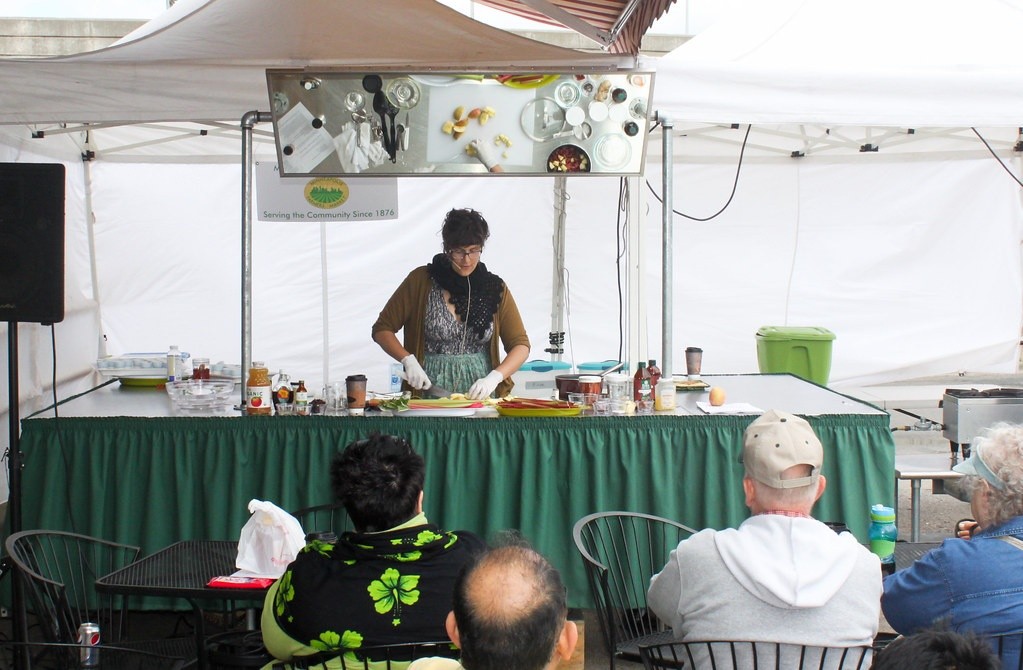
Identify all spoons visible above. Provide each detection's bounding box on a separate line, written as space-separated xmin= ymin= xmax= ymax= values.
xmin=373 ymin=91 xmax=399 ymax=163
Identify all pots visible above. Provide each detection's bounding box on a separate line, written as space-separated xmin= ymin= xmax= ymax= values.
xmin=555 ymin=361 xmax=625 ymax=401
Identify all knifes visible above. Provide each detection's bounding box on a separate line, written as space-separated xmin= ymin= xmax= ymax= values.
xmin=402 ymin=112 xmax=409 ymax=151
xmin=396 ymin=369 xmax=454 ymax=400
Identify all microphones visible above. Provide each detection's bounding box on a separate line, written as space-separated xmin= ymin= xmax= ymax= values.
xmin=448 ymin=257 xmax=461 ymax=270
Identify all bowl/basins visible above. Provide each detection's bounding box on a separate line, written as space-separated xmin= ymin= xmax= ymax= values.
xmin=569 ymin=393 xmax=653 ymax=415
xmin=520 ymin=75 xmax=645 ymax=173
xmin=309 ymin=402 xmax=327 ymax=416
xmin=96 ymin=355 xmax=167 ymax=368
xmin=214 ymin=362 xmax=241 ymax=377
xmin=275 ymin=402 xmax=294 ymax=416
xmin=294 ymin=404 xmax=313 ymax=415
xmin=166 ymin=379 xmax=236 ymax=411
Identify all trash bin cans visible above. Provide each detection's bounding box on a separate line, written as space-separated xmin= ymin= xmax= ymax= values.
xmin=755 ymin=325 xmax=837 ymax=387
xmin=203 ymin=631 xmax=275 ymax=670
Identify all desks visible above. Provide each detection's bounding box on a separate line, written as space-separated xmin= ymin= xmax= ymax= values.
xmin=93 ymin=539 xmax=267 ymax=670
xmin=895 ymin=451 xmax=970 ymax=545
xmin=862 ymin=542 xmax=944 ymax=578
xmin=0 ymin=371 xmax=893 ymax=639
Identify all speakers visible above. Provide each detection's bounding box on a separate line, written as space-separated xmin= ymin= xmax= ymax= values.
xmin=0 ymin=163 xmax=66 ymax=326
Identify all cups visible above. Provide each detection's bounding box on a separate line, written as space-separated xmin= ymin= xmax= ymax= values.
xmin=577 ymin=376 xmax=602 ymax=395
xmin=345 ymin=375 xmax=367 ymax=409
xmin=344 ymin=91 xmax=365 ymax=112
xmin=385 ymin=76 xmax=419 ymax=109
xmin=685 ymin=347 xmax=703 ymax=380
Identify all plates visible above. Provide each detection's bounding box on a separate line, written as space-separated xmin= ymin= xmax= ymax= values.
xmin=119 ymin=377 xmax=167 ymax=386
xmin=495 ymin=406 xmax=583 ymax=416
xmin=393 ymin=408 xmax=477 ymax=417
xmin=605 ymin=359 xmax=676 ymax=410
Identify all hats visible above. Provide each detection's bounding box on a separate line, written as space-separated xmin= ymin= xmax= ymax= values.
xmin=952 ymin=441 xmax=1007 ymax=494
xmin=737 ymin=408 xmax=824 ymax=489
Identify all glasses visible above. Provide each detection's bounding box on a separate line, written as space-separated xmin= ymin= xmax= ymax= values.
xmin=444 ymin=246 xmax=482 ymax=260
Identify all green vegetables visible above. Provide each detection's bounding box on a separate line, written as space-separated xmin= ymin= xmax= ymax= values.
xmin=375 ymin=397 xmax=489 ymax=412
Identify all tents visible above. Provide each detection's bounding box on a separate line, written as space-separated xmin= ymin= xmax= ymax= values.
xmin=0 ymin=0 xmax=1023 ymax=508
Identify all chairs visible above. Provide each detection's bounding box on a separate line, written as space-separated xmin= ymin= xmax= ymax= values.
xmin=637 ymin=639 xmax=884 ymax=670
xmin=874 ymin=630 xmax=1023 ymax=670
xmin=4 ymin=529 xmax=198 ymax=670
xmin=0 ymin=641 xmax=186 ymax=670
xmin=289 ymin=504 xmax=348 ymax=533
xmin=572 ymin=509 xmax=699 ymax=670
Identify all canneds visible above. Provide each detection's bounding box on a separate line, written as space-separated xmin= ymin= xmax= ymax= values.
xmin=246 ymin=368 xmax=271 ymax=414
xmin=77 ymin=623 xmax=100 ymax=667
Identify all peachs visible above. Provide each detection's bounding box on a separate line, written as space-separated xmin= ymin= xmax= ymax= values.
xmin=709 ymin=386 xmax=725 ymax=407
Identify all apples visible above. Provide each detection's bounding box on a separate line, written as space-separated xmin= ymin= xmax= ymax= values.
xmin=251 ymin=397 xmax=262 ymax=407
xmin=548 ymin=147 xmax=589 ymax=173
xmin=450 ymin=393 xmax=471 ymax=399
xmin=442 ymin=105 xmax=496 ymax=140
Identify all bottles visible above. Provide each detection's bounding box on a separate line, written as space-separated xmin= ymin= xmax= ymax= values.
xmin=245 ymin=362 xmax=308 ymax=416
xmin=168 ymin=338 xmax=213 ymax=379
xmin=869 ymin=504 xmax=896 ymax=575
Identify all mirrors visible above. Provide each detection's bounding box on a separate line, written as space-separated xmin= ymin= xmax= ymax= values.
xmin=265 ymin=66 xmax=656 ymax=177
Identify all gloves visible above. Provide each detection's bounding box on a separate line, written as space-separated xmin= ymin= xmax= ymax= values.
xmin=400 ymin=354 xmax=431 ymax=390
xmin=467 ymin=370 xmax=504 ymax=401
xmin=469 ymin=139 xmax=501 ymax=173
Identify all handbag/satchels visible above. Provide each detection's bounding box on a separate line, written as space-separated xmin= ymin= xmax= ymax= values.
xmin=235 ymin=498 xmax=306 ymax=578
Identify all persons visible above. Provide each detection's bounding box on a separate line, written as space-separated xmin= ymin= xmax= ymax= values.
xmin=409 ymin=529 xmax=580 ymax=670
xmin=470 ymin=139 xmax=504 ymax=173
xmin=373 ymin=208 xmax=531 ymax=400
xmin=881 ymin=421 xmax=1023 ymax=670
xmin=648 ymin=411 xmax=884 ymax=670
xmin=261 ymin=430 xmax=492 ymax=670
xmin=870 ymin=616 xmax=1001 ymax=670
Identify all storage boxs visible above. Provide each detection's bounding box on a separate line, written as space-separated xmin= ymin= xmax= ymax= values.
xmin=755 ymin=325 xmax=836 ymax=386
